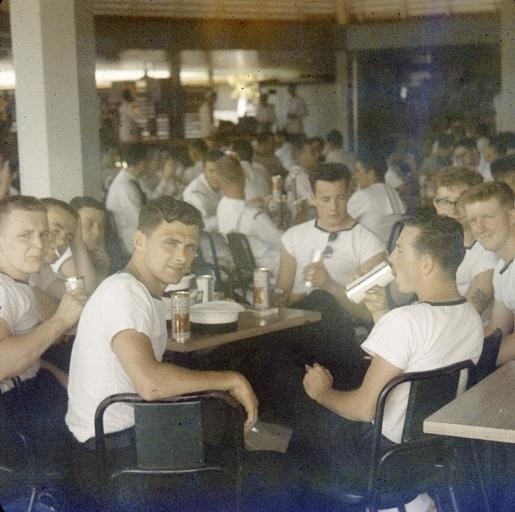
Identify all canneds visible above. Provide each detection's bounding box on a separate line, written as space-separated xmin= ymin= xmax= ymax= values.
xmin=272 ymin=175 xmax=282 ymax=199
xmin=285 ymin=176 xmax=296 ymax=200
xmin=344 ymin=260 xmax=396 ymax=304
xmin=63 ymin=275 xmax=87 ymax=336
xmin=171 ymin=291 xmax=191 ymax=343
xmin=195 ymin=274 xmax=214 ymax=305
xmin=301 ymin=247 xmax=326 ymax=288
xmin=253 ymin=268 xmax=271 ymax=310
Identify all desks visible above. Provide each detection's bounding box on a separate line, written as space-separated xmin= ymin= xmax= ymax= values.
xmin=165 ymin=305 xmax=322 ymax=353
xmin=424 ymin=356 xmax=514 ymax=444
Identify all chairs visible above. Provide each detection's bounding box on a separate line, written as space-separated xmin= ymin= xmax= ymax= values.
xmin=93 ymin=392 xmax=245 ymax=512
xmin=184 ymin=230 xmax=258 ymax=305
xmin=103 ymin=210 xmax=128 ymax=276
xmin=0 ymin=377 xmax=68 ymax=512
xmin=386 ymin=219 xmax=419 ymax=309
xmin=302 ymin=327 xmax=503 ymax=512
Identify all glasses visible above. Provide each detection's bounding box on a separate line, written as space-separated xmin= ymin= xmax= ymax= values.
xmin=320 ymin=229 xmax=339 ymax=258
